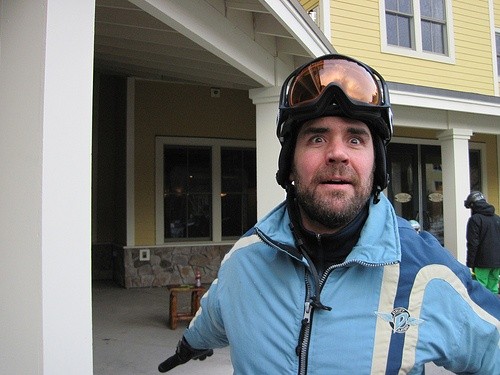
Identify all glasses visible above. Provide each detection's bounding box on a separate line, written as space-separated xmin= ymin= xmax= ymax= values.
xmin=282 ymin=59 xmax=382 ymax=107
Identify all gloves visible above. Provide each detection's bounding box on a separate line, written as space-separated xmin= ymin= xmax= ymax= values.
xmin=158 ymin=335 xmax=213 ymax=373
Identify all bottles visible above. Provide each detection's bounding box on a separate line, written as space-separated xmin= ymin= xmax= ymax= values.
xmin=195 ymin=271 xmax=202 ymax=288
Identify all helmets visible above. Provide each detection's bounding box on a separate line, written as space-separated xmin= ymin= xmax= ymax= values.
xmin=464 ymin=191 xmax=486 ymax=208
xmin=275 ymin=53 xmax=394 ymax=192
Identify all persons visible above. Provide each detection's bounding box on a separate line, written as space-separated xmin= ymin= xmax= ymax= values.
xmin=158 ymin=52 xmax=500 ymax=375
xmin=464 ymin=191 xmax=500 ymax=294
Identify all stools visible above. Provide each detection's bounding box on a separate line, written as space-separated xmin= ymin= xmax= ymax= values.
xmin=167 ymin=284 xmax=204 ymax=329
